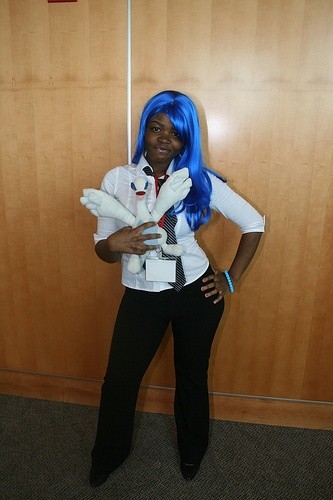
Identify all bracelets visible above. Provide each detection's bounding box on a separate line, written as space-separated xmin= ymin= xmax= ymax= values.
xmin=224 ymin=270 xmax=234 ymax=294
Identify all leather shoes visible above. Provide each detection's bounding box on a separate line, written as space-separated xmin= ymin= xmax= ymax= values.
xmin=180 ymin=461 xmax=199 ymax=482
xmin=90 ymin=469 xmax=106 ymax=486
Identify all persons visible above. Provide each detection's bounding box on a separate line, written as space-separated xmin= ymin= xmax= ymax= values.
xmin=91 ymin=90 xmax=265 ymax=487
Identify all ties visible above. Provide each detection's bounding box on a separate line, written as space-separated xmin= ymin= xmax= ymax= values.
xmin=142 ymin=166 xmax=186 ymax=292
